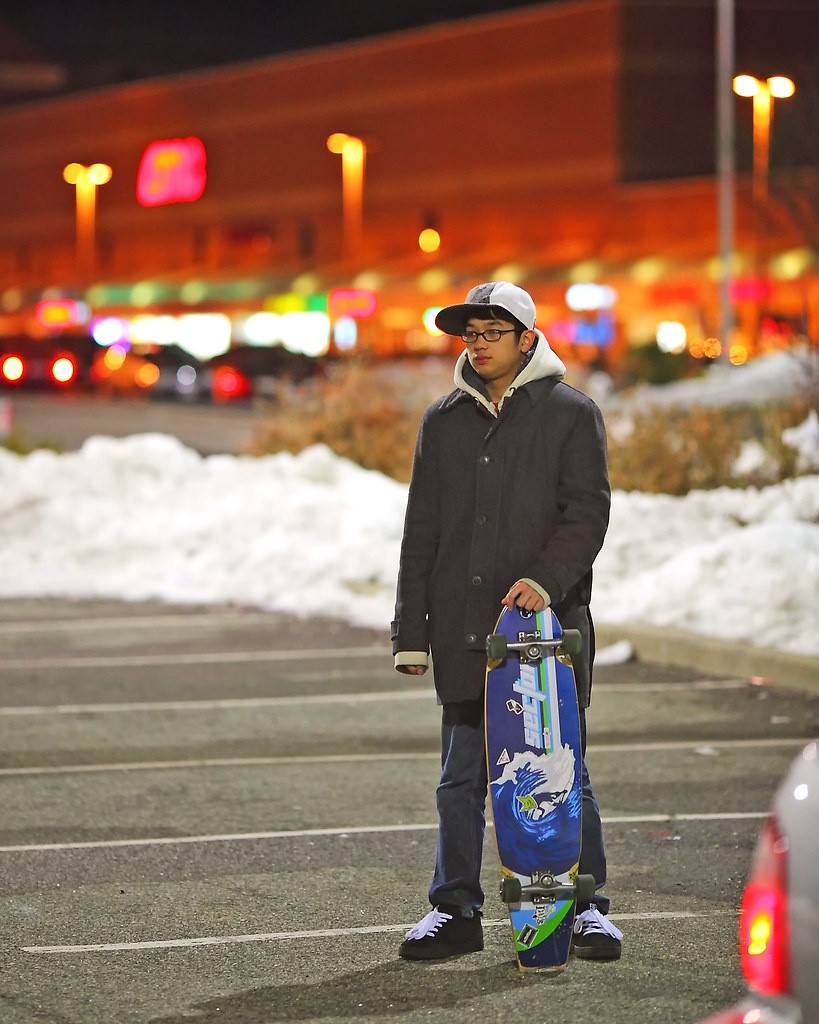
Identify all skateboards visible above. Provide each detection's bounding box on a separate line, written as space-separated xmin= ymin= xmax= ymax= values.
xmin=482 ymin=593 xmax=587 ymax=974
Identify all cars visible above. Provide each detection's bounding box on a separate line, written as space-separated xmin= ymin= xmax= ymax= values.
xmin=1 ymin=330 xmax=103 ymax=390
xmin=91 ymin=342 xmax=198 ymax=403
xmin=694 ymin=731 xmax=818 ymax=1024
xmin=193 ymin=347 xmax=321 ymax=405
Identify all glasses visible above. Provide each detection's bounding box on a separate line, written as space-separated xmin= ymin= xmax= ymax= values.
xmin=460 ymin=329 xmax=515 ymax=343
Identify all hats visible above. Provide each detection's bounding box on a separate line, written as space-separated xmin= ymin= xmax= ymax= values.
xmin=435 ymin=281 xmax=536 ymax=336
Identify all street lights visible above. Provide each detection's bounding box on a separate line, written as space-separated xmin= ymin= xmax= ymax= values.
xmin=732 ymin=73 xmax=795 ymax=322
xmin=327 ymin=134 xmax=365 ymax=261
xmin=62 ymin=162 xmax=111 ymax=294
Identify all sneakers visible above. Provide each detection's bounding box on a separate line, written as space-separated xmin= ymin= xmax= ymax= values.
xmin=572 ymin=905 xmax=623 ymax=959
xmin=397 ymin=906 xmax=485 ymax=959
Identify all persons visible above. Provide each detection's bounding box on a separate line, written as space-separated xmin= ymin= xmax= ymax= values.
xmin=388 ymin=283 xmax=628 ymax=962
